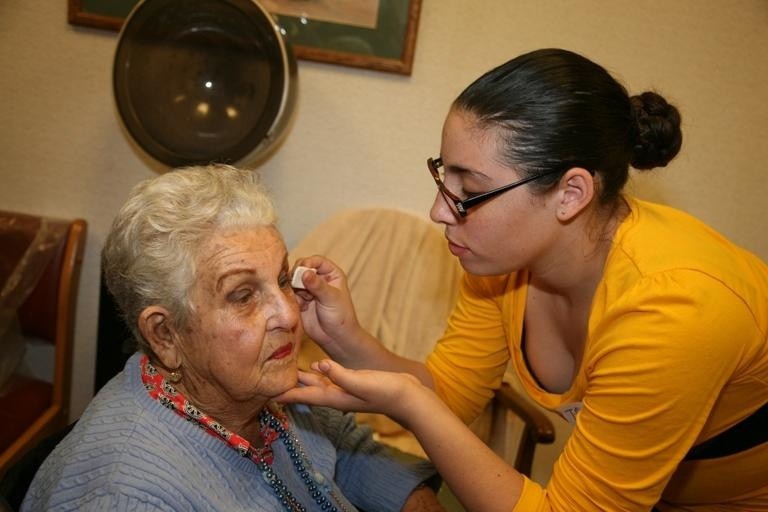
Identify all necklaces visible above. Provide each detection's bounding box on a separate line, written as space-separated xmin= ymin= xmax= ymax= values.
xmin=254 ymin=406 xmax=348 ymax=511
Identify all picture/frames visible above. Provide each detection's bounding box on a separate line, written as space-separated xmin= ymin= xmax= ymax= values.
xmin=65 ymin=0 xmax=428 ymax=76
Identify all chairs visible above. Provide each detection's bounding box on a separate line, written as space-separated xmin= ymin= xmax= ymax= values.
xmin=282 ymin=208 xmax=556 ymax=481
xmin=0 ymin=210 xmax=91 ymax=490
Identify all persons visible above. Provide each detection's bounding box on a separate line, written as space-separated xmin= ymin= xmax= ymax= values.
xmin=22 ymin=165 xmax=446 ymax=512
xmin=272 ymin=47 xmax=767 ymax=512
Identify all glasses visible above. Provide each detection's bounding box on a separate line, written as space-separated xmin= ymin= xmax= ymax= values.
xmin=426 ymin=157 xmax=561 ymax=216
